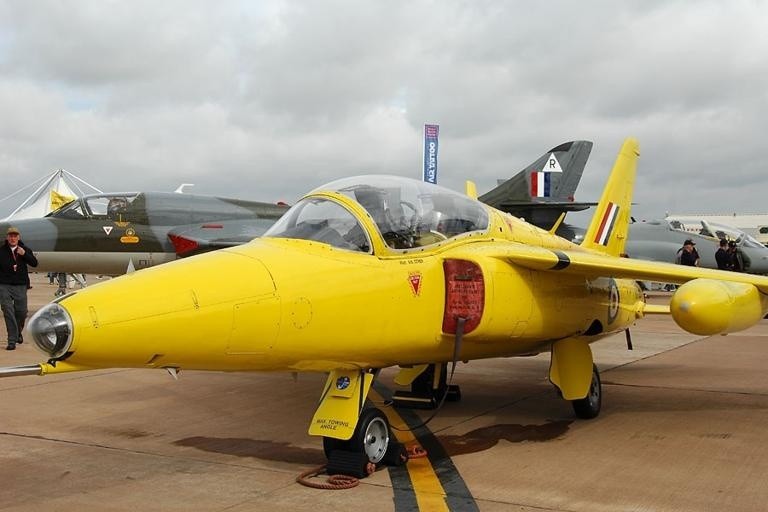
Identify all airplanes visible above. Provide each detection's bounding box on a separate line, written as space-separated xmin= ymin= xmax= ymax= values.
xmin=1 ymin=139 xmax=600 ymax=287
xmin=0 ymin=133 xmax=767 ymax=477
xmin=564 ymin=214 xmax=768 ymax=276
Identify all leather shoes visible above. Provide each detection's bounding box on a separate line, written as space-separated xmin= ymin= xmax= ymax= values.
xmin=18 ymin=333 xmax=23 ymax=344
xmin=7 ymin=343 xmax=15 ymax=349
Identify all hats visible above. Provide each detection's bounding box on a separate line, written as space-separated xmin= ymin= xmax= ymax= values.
xmin=7 ymin=228 xmax=20 ymax=234
xmin=720 ymin=239 xmax=737 ymax=247
xmin=684 ymin=240 xmax=697 ymax=247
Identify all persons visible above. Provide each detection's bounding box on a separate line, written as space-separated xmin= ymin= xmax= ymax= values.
xmin=679 ymin=239 xmax=700 ymax=266
xmin=0 ymin=226 xmax=38 ymax=350
xmin=714 ymin=238 xmax=737 ymax=270
xmin=674 ymin=237 xmax=696 ymax=264
xmin=726 ymin=240 xmax=741 ymax=272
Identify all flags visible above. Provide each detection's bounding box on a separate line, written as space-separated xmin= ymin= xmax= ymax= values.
xmin=49 ymin=189 xmax=75 ymax=214
xmin=423 ymin=122 xmax=439 ymax=185
